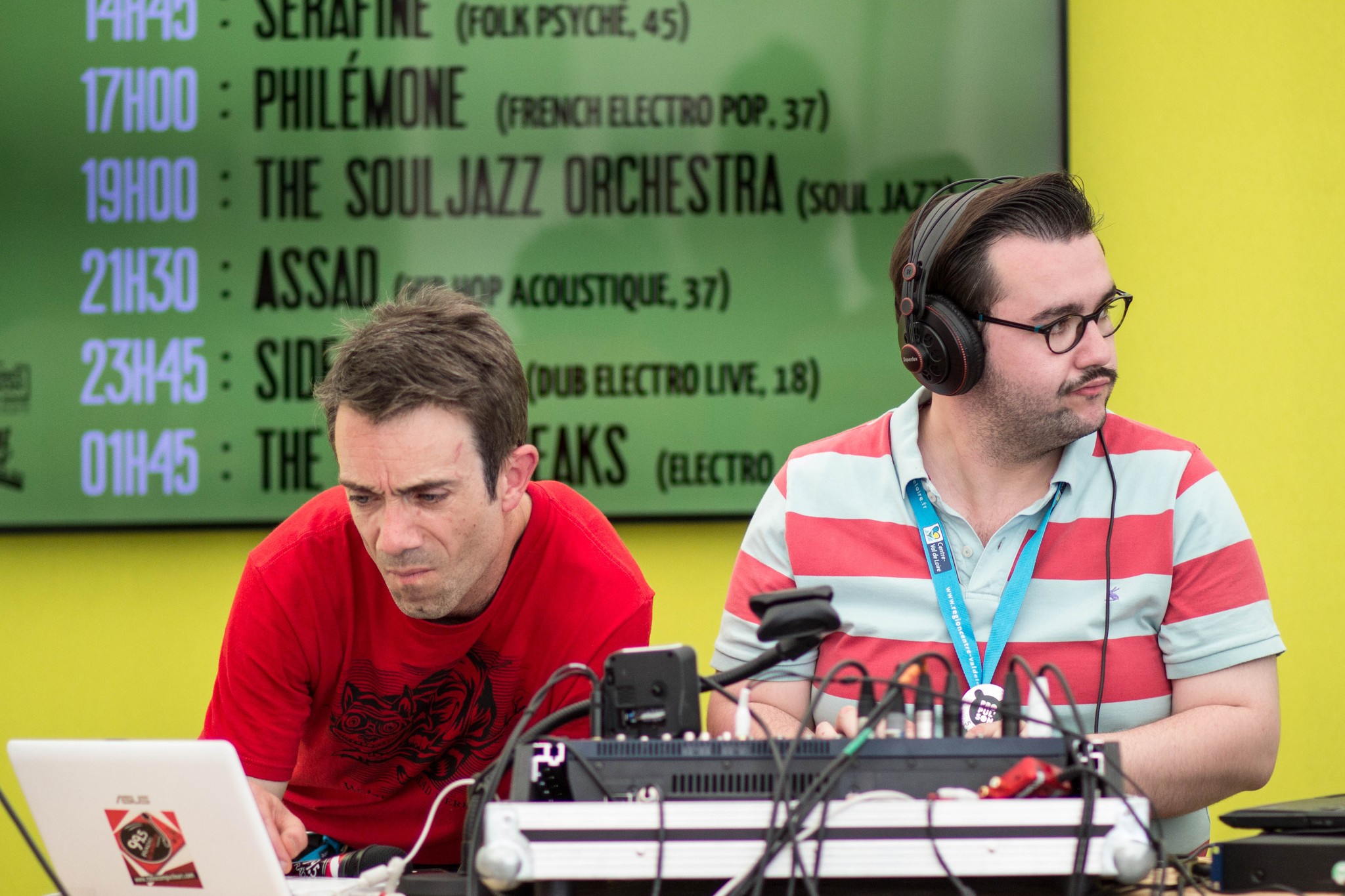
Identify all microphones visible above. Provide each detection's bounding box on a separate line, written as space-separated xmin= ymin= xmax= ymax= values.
xmin=284 ymin=845 xmax=415 ymax=878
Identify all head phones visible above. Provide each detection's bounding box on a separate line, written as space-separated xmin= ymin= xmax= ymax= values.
xmin=897 ymin=176 xmax=1026 ymax=397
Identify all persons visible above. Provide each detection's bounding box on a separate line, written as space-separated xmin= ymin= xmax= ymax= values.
xmin=198 ymin=285 xmax=655 ymax=879
xmin=706 ymin=170 xmax=1285 ymax=866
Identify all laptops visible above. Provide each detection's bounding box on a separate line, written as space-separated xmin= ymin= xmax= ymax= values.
xmin=1218 ymin=791 xmax=1345 ymax=834
xmin=5 ymin=738 xmax=384 ymax=896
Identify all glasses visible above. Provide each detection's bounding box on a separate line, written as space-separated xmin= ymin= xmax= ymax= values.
xmin=966 ymin=288 xmax=1133 ymax=354
